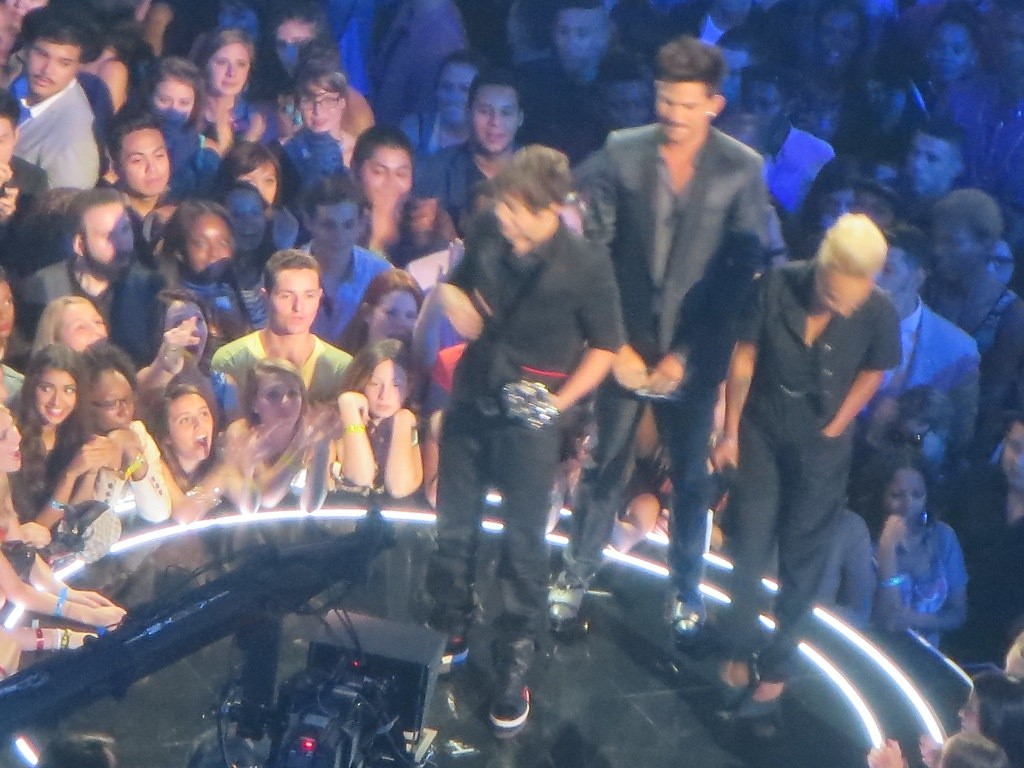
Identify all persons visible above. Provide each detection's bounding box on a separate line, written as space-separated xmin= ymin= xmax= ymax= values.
xmin=3 ymin=0 xmax=1024 ymax=768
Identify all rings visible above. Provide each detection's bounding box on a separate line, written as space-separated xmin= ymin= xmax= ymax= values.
xmin=670 ymin=381 xmax=675 ymax=385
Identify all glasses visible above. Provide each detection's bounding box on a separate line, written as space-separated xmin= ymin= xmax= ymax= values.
xmin=296 ymin=95 xmax=342 ymax=111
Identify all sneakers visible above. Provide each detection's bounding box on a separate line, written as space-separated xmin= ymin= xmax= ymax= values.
xmin=488 ymin=680 xmax=529 ymax=728
xmin=424 ymin=620 xmax=468 ymax=663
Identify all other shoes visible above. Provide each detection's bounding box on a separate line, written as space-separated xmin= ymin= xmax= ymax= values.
xmin=712 ymin=657 xmax=759 ymax=725
xmin=744 ymin=694 xmax=783 ymax=748
xmin=671 ymin=591 xmax=705 ymax=645
xmin=547 ymin=567 xmax=586 ymax=632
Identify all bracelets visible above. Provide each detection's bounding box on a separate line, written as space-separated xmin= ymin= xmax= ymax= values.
xmin=36 ymin=627 xmax=43 ymax=650
xmin=185 ymin=488 xmax=223 ymax=509
xmin=49 ymin=498 xmax=67 ymax=511
xmin=344 ymin=424 xmax=368 ymax=434
xmin=125 ymin=452 xmax=144 ymax=479
xmin=280 ymin=455 xmax=307 ymax=472
xmin=54 ymin=585 xmax=68 ymax=619
xmin=881 ymin=574 xmax=904 ymax=588
xmin=56 ymin=627 xmax=70 ymax=651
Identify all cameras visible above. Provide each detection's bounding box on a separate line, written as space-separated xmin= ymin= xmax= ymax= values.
xmin=896 ymin=384 xmax=930 ymax=420
